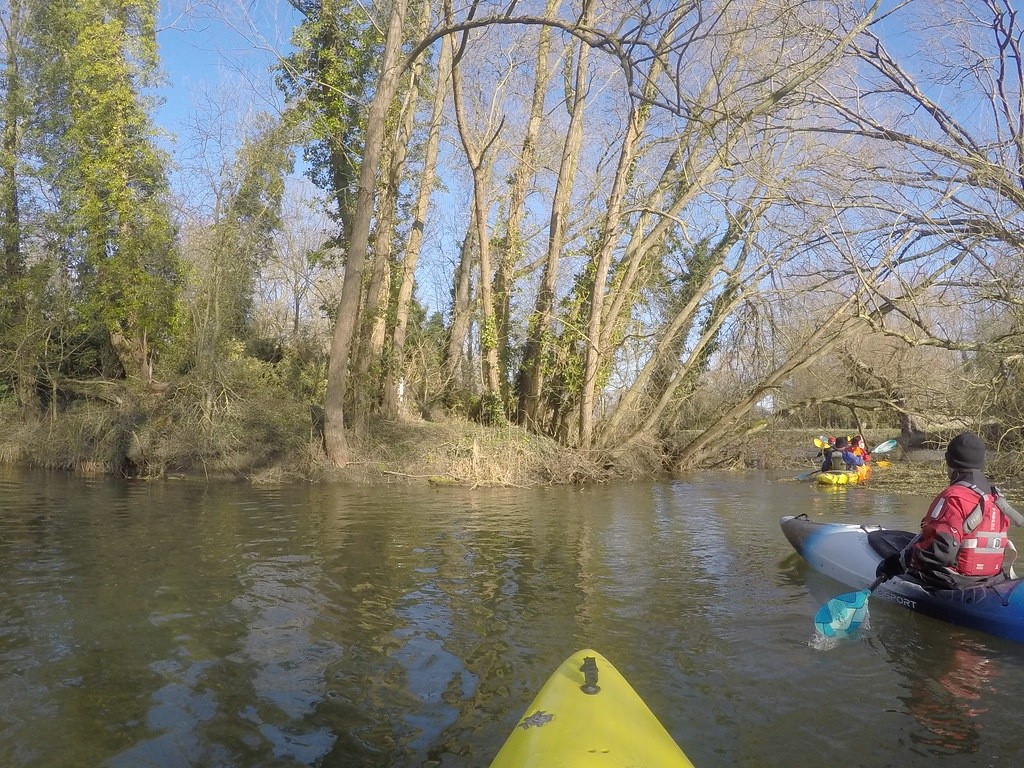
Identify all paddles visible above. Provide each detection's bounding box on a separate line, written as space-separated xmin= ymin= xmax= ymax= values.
xmin=796 ymin=435 xmax=896 ymax=483
xmin=813 ymin=531 xmax=925 ymax=643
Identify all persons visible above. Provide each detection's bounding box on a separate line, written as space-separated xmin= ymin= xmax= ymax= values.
xmin=876 ymin=431 xmax=1011 ymax=589
xmin=817 ymin=435 xmax=870 ymax=472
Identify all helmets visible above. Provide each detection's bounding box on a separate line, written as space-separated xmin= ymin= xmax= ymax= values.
xmin=828 ymin=438 xmax=834 ymax=444
xmin=844 ymin=435 xmax=851 ymax=441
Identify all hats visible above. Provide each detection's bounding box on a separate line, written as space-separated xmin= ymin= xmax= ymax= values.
xmin=835 ymin=437 xmax=847 ymax=447
xmin=851 ymin=438 xmax=860 ymax=441
xmin=946 ymin=433 xmax=986 ymax=469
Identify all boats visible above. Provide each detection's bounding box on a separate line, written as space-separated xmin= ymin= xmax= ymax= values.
xmin=777 ymin=512 xmax=1024 ymax=646
xmin=485 ymin=643 xmax=716 ymax=767
xmin=816 ymin=465 xmax=871 ymax=492
